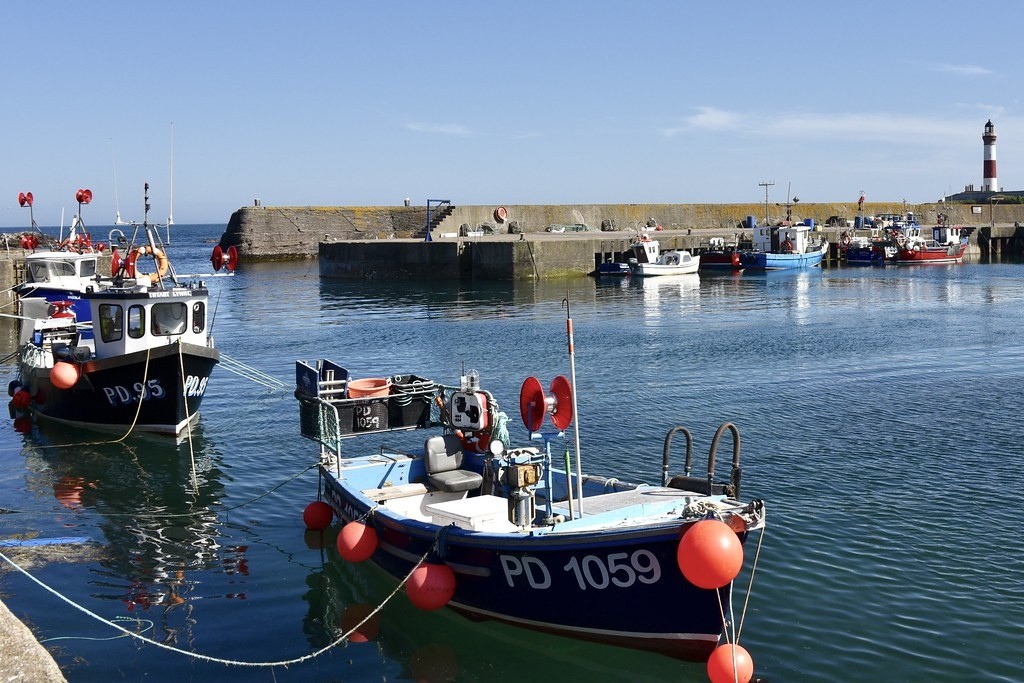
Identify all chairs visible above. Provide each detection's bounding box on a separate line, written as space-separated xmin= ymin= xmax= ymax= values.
xmin=424 ymin=433 xmax=484 ymax=493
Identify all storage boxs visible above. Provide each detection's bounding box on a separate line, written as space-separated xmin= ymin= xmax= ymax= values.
xmin=298 ymin=399 xmax=356 ymax=437
xmin=384 ymin=374 xmax=433 ymax=428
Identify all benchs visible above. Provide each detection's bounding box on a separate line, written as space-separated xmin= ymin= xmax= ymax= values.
xmin=362 ymin=483 xmax=440 ymax=505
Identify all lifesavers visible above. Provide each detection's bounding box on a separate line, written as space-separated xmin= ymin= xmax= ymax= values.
xmin=843 ymin=235 xmax=851 ymax=246
xmin=59 ymin=237 xmax=69 ymax=249
xmin=780 ymin=240 xmax=792 ymax=253
xmin=452 ymin=394 xmax=495 ymax=455
xmin=68 ymin=240 xmax=92 ymax=253
xmin=641 ymin=234 xmax=649 ymax=241
xmin=134 ymin=245 xmax=168 ymax=283
xmin=497 ymin=207 xmax=506 ymax=219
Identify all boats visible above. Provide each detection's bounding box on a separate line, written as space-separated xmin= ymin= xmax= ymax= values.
xmin=292 ymin=356 xmax=767 ymax=664
xmin=599 ymin=233 xmax=701 ymax=278
xmin=12 ymin=217 xmax=222 ymax=437
xmin=701 ymin=236 xmax=749 ymax=268
xmin=842 ymin=215 xmax=970 ymax=266
xmin=734 ymin=196 xmax=830 ymax=271
